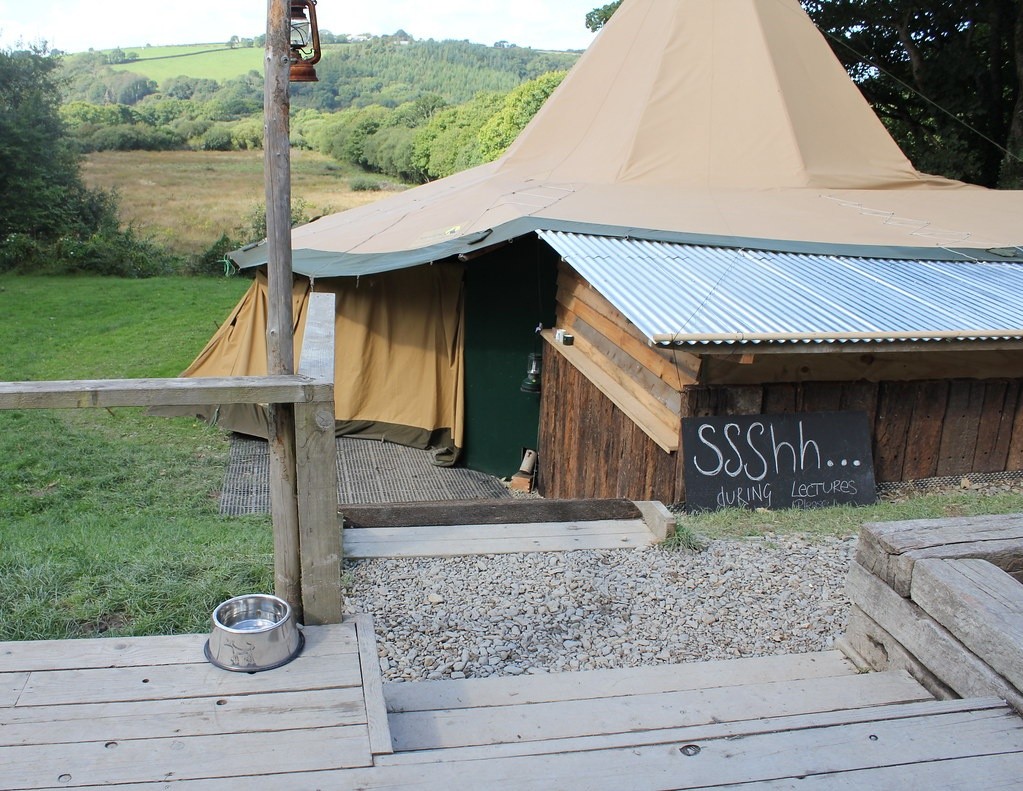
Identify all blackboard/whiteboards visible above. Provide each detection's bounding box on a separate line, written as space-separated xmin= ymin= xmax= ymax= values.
xmin=681 ymin=408 xmax=876 ymax=518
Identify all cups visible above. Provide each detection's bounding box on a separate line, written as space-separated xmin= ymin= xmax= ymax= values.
xmin=519 ymin=449 xmax=536 ymax=475
xmin=552 ymin=327 xmax=573 ymax=345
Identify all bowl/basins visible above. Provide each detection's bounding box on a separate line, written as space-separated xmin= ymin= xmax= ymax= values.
xmin=204 ymin=593 xmax=306 ymax=673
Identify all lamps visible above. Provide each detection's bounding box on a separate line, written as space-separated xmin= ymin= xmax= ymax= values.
xmin=519 ymin=354 xmax=542 ymax=395
xmin=287 ymin=0 xmax=324 ymax=82
